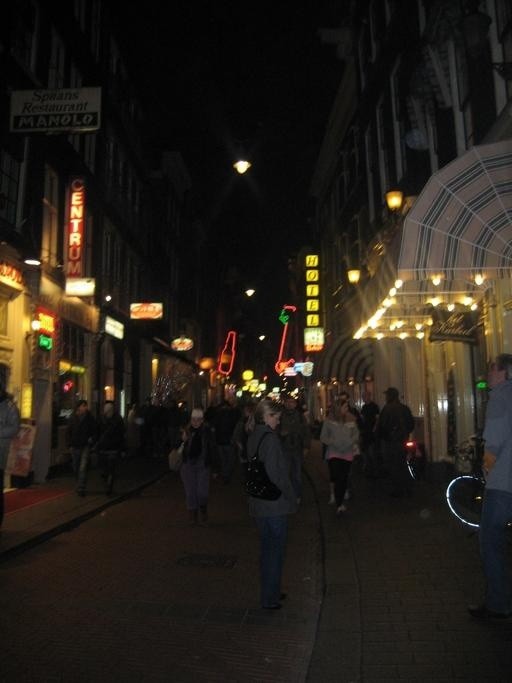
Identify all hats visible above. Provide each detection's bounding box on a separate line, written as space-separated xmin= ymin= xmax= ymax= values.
xmin=382 ymin=387 xmax=399 ymax=396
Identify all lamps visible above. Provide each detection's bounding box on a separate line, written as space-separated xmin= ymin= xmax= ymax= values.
xmin=384 ymin=188 xmax=404 ymax=211
xmin=345 ymin=265 xmax=361 ymax=285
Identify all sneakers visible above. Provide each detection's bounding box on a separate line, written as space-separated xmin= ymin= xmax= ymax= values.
xmin=467 ymin=605 xmax=512 ymax=626
xmin=328 ymin=489 xmax=350 ymax=504
xmin=337 ymin=504 xmax=346 ymax=513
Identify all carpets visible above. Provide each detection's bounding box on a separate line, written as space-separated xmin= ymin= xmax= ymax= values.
xmin=0 ymin=484 xmax=74 ymax=518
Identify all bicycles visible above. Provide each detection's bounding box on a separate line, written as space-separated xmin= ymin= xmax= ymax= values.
xmin=403 ymin=439 xmax=422 ymax=480
xmin=445 ymin=433 xmax=489 ymax=528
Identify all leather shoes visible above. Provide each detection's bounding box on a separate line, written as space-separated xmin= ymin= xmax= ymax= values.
xmin=262 ymin=592 xmax=289 ymax=609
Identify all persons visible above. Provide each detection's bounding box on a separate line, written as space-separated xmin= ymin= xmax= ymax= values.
xmin=479 ymin=354 xmax=512 ymax=624
xmin=0 ymin=363 xmax=21 ymax=525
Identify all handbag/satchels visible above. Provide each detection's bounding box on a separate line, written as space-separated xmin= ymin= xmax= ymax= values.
xmin=167 ymin=448 xmax=184 ymax=472
xmin=241 ymin=456 xmax=281 ymax=501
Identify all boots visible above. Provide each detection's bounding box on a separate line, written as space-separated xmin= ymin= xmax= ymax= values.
xmin=199 ymin=502 xmax=209 ymax=522
xmin=187 ymin=506 xmax=199 ymax=525
